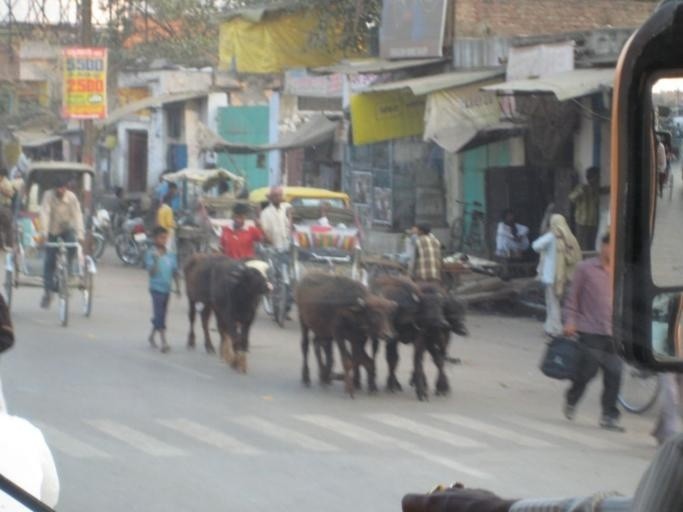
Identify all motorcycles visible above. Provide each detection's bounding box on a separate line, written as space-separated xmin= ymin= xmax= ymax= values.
xmin=90 ymin=206 xmax=110 ymax=259
xmin=114 ymin=203 xmax=149 ymax=272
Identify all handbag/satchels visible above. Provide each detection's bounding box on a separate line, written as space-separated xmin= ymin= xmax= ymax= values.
xmin=542 ymin=335 xmax=583 ymax=381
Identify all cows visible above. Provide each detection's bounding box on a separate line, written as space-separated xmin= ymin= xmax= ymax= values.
xmin=182 ymin=252 xmax=275 ymax=375
xmin=296 ymin=272 xmax=468 ymax=402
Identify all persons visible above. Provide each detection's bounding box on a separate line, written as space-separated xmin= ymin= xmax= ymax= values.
xmin=0 ymin=289 xmax=62 ymax=511
xmin=497 ymin=167 xmax=602 ymax=339
xmin=144 ymin=155 xmax=346 ymax=353
xmin=654 ymin=136 xmax=670 ymax=200
xmin=401 ymin=429 xmax=681 ymax=511
xmin=35 ymin=175 xmax=84 ymax=308
xmin=407 ymin=221 xmax=442 ymax=285
xmin=562 ymin=235 xmax=622 ymax=431
xmin=110 ymin=186 xmax=138 ymax=228
xmin=1 ymin=168 xmax=26 ymax=246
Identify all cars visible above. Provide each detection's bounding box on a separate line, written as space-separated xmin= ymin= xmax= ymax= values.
xmin=249 ymin=184 xmax=360 ymax=250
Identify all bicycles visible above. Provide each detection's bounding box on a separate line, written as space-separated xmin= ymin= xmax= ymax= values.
xmin=260 ymin=245 xmax=294 ymax=326
xmin=449 ymin=196 xmax=496 ymax=261
xmin=614 ymin=350 xmax=660 ymax=416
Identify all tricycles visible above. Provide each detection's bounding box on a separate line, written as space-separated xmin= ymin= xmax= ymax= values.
xmin=655 ymin=128 xmax=674 ymax=200
xmin=3 ymin=159 xmax=98 ymax=325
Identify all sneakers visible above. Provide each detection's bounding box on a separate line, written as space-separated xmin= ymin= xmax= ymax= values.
xmin=601 ymin=414 xmax=625 ymax=433
xmin=561 ymin=388 xmax=576 ymax=420
